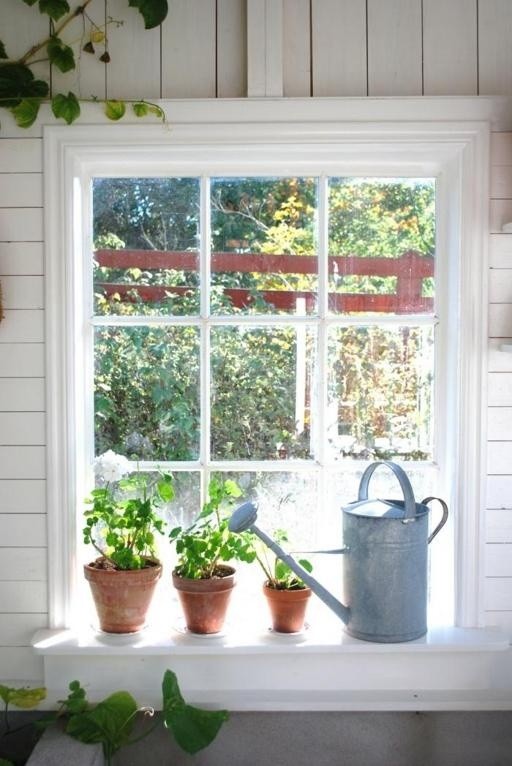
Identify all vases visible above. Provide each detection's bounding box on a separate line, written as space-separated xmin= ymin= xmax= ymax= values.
xmin=83 ymin=556 xmax=163 ymax=633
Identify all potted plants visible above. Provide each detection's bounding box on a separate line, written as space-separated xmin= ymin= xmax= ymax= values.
xmin=241 ymin=527 xmax=312 ymax=634
xmin=168 ymin=470 xmax=257 ymax=634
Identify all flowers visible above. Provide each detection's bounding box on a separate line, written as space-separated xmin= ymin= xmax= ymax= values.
xmin=81 ymin=449 xmax=174 ymax=571
